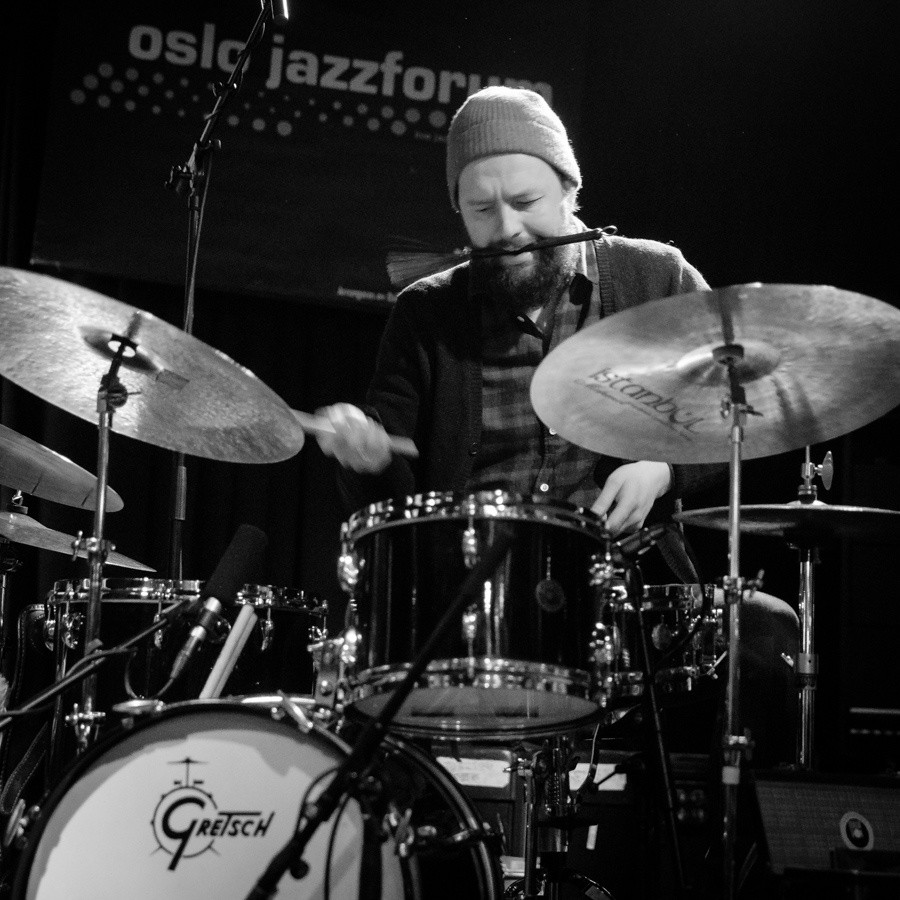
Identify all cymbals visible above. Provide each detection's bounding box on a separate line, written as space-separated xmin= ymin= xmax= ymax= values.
xmin=0 ymin=510 xmax=157 ymax=573
xmin=529 ymin=283 xmax=900 ymax=465
xmin=0 ymin=264 xmax=306 ymax=465
xmin=0 ymin=424 xmax=125 ymax=512
xmin=671 ymin=501 xmax=900 ymax=546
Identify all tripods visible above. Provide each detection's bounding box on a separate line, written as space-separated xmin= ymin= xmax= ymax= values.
xmin=498 ymin=731 xmax=615 ymax=900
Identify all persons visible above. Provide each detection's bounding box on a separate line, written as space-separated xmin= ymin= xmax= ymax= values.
xmin=309 ymin=86 xmax=800 ymax=900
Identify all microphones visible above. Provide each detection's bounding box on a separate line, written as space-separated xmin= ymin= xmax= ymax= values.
xmin=170 ymin=596 xmax=222 ymax=682
xmin=620 ymin=525 xmax=665 ymax=558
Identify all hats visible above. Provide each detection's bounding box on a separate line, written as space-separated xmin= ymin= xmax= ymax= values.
xmin=447 ymin=87 xmax=582 ymax=212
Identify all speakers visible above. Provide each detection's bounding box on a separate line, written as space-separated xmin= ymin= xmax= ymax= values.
xmin=732 ymin=778 xmax=899 ymax=900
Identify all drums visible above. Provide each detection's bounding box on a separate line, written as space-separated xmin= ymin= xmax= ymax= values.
xmin=17 ymin=601 xmax=57 ymax=748
xmin=8 ymin=694 xmax=504 ymax=900
xmin=336 ymin=489 xmax=616 ymax=731
xmin=58 ymin=579 xmax=328 ymax=731
xmin=582 ymin=584 xmax=724 ymax=696
